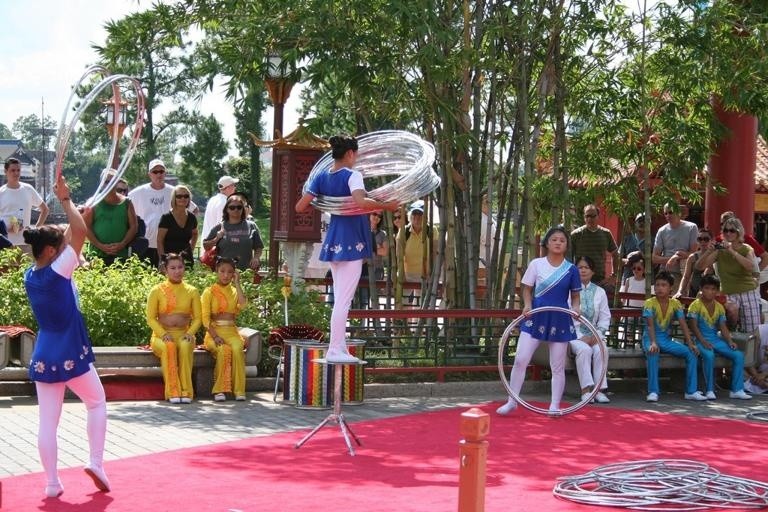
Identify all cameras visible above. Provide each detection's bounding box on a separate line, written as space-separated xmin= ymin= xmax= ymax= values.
xmin=715 ymin=242 xmax=726 ymax=249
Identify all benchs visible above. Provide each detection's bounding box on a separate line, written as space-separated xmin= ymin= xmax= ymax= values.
xmin=531 ymin=333 xmax=754 ymax=394
xmin=21 ymin=324 xmax=262 ymax=399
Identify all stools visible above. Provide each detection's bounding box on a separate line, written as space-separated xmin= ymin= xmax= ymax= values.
xmin=293 ymin=357 xmax=369 ymax=457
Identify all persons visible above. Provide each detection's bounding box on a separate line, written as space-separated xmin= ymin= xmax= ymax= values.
xmin=325 ymin=207 xmax=440 ymax=335
xmin=0 ymin=219 xmax=16 ymax=253
xmin=55 ymin=223 xmax=89 ymax=266
xmin=24 ymin=173 xmax=112 ymax=499
xmin=200 ymin=257 xmax=249 ymax=402
xmin=295 ymin=136 xmax=401 ymax=363
xmin=145 ymin=253 xmax=202 ymax=404
xmin=0 ymin=156 xmax=48 ymax=249
xmin=227 ymin=192 xmax=249 ymax=217
xmin=200 ymin=174 xmax=240 ymax=257
xmin=77 ymin=160 xmax=198 ymax=268
xmin=202 ymin=194 xmax=264 ymax=270
xmin=495 ymin=202 xmax=768 ymax=414
xmin=245 ymin=203 xmax=255 ymax=222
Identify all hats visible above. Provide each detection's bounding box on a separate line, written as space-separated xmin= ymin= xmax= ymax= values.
xmin=149 ymin=159 xmax=165 ymax=170
xmin=410 ymin=203 xmax=424 ymax=214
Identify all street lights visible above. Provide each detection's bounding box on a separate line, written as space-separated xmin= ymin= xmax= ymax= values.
xmin=260 ymin=41 xmax=300 ymax=285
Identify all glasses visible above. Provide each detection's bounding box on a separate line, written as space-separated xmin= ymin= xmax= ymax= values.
xmin=392 ymin=215 xmax=402 ymax=220
xmin=372 ymin=212 xmax=383 ymax=219
xmin=106 ymin=170 xmax=244 ymax=209
xmin=584 ymin=210 xmax=737 ymax=271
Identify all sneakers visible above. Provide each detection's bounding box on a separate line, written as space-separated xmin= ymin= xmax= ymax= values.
xmin=326 ymin=352 xmax=357 ymax=363
xmin=169 ymin=396 xmax=191 ymax=403
xmin=236 ymin=394 xmax=246 ymax=401
xmin=47 ymin=483 xmax=63 ymax=498
xmin=214 ymin=393 xmax=226 ymax=401
xmin=496 ymin=389 xmax=752 ymax=415
xmin=86 ymin=462 xmax=110 ymax=490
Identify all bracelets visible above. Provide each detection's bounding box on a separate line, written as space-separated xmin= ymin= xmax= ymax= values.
xmin=60 ymin=196 xmax=70 ymax=202
xmin=236 ymin=280 xmax=240 ymax=284
xmin=214 ymin=335 xmax=218 ymax=339
